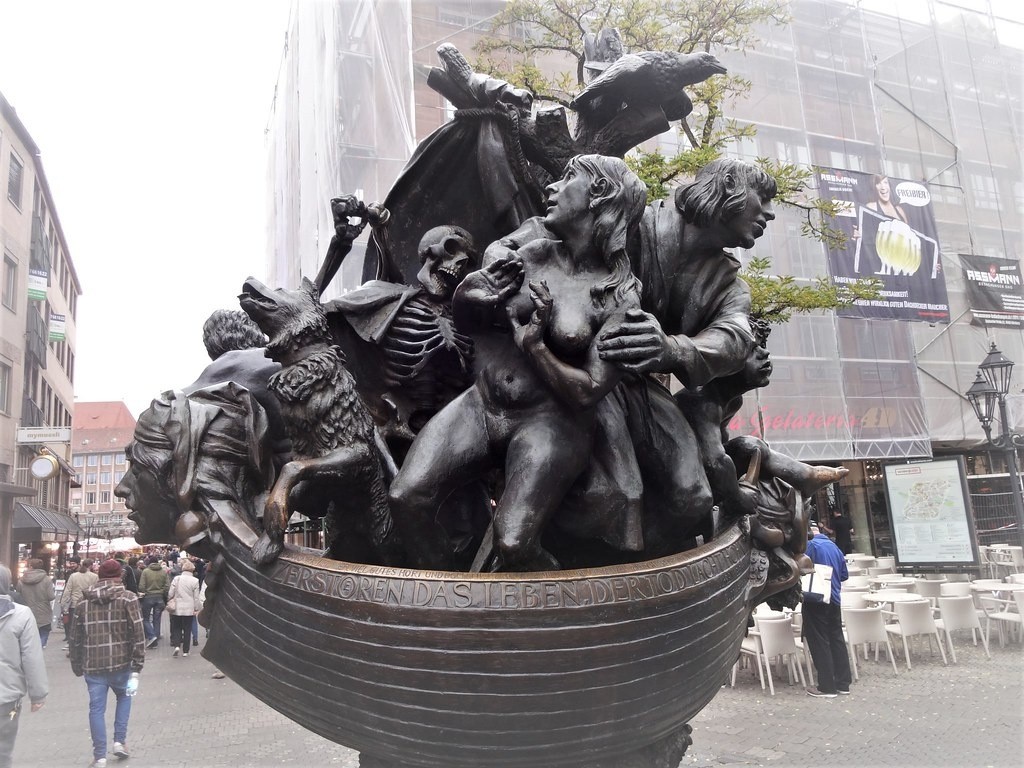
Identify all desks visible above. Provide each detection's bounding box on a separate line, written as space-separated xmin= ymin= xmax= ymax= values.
xmin=971 ymin=583 xmax=1024 ymax=645
xmin=983 ymin=546 xmax=1023 ymax=579
xmin=868 ymin=578 xmax=915 ymax=582
xmin=862 ymin=592 xmax=922 ymax=661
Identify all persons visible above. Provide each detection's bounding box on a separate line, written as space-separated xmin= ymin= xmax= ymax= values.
xmin=851 ymin=174 xmax=909 ymax=241
xmin=0 ymin=545 xmax=209 ymax=768
xmin=801 ymin=508 xmax=854 ymax=698
xmin=113 ymin=152 xmax=849 ymax=571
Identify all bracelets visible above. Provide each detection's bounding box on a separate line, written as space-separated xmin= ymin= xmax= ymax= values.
xmin=821 ymin=525 xmax=825 ymax=529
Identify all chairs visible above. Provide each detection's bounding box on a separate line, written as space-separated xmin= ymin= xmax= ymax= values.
xmin=728 ymin=543 xmax=1024 ymax=696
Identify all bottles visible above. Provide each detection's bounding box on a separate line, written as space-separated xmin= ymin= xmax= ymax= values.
xmin=125 ymin=669 xmax=139 ymax=697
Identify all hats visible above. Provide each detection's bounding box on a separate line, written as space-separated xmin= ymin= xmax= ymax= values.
xmin=809 ymin=520 xmax=818 ymax=527
xmin=99 ymin=559 xmax=121 ymax=578
xmin=70 ymin=556 xmax=81 ymax=564
xmin=31 ymin=560 xmax=43 ymax=569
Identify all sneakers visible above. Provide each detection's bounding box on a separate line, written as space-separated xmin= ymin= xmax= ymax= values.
xmin=113 ymin=742 xmax=130 ymax=758
xmin=88 ymin=758 xmax=106 ymax=768
xmin=836 ymin=689 xmax=850 ymax=695
xmin=807 ymin=687 xmax=837 ymax=698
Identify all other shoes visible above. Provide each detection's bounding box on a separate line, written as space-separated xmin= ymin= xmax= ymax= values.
xmin=212 ymin=671 xmax=225 ymax=679
xmin=63 ymin=638 xmax=68 ymax=641
xmin=193 ymin=640 xmax=198 ymax=646
xmin=145 ymin=637 xmax=158 ymax=649
xmin=172 ymin=647 xmax=179 ymax=656
xmin=183 ymin=653 xmax=190 ymax=657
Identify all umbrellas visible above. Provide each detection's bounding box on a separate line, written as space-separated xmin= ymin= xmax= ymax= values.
xmin=45 ymin=537 xmax=171 ymax=556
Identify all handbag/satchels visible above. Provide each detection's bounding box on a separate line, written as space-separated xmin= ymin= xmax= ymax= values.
xmin=801 ymin=540 xmax=833 ymax=604
xmin=165 ymin=576 xmax=180 ymax=612
xmin=137 ymin=592 xmax=146 ymax=599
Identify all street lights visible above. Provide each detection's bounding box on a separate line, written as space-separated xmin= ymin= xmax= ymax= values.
xmin=85 ymin=510 xmax=96 ymax=559
xmin=966 ymin=342 xmax=1024 ymax=558
